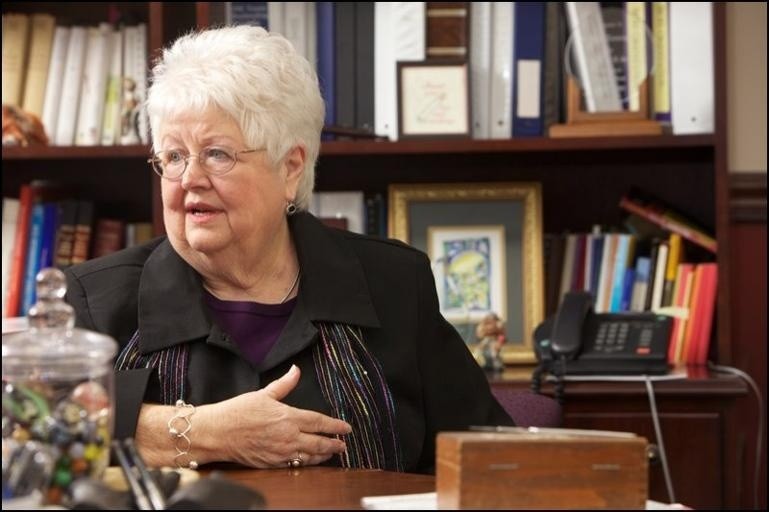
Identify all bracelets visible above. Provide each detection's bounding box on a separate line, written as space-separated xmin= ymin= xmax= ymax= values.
xmin=166 ymin=398 xmax=200 ymax=471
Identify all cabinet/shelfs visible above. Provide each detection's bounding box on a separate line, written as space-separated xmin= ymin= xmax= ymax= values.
xmin=2 ymin=2 xmax=749 ymax=510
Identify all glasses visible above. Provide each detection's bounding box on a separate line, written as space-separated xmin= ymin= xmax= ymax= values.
xmin=147 ymin=144 xmax=268 ymax=180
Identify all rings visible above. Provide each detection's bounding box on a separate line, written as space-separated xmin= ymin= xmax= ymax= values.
xmin=286 ymin=447 xmax=305 ymax=470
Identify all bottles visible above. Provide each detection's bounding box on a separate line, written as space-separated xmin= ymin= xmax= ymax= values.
xmin=1 ymin=270 xmax=117 ymax=512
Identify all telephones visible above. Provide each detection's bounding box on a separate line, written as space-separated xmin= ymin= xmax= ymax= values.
xmin=532 ymin=292 xmax=675 ymax=375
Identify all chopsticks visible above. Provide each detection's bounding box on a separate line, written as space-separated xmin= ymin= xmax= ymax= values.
xmin=111 ymin=436 xmax=167 ymax=512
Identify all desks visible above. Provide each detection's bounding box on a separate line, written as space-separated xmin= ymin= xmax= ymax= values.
xmin=205 ymin=468 xmax=435 ymax=510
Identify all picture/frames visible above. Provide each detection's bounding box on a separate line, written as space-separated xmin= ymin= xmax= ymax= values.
xmin=396 ymin=59 xmax=471 ymax=140
xmin=388 ymin=182 xmax=544 ymax=365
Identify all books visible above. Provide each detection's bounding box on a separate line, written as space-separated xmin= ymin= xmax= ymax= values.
xmin=1 ymin=15 xmax=153 ymax=145
xmin=554 ymin=193 xmax=721 ymax=367
xmin=307 ymin=189 xmax=388 ymax=239
xmin=198 ymin=0 xmax=716 ymax=140
xmin=0 ymin=182 xmax=152 ymax=318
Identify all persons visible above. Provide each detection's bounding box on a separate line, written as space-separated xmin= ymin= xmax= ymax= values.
xmin=57 ymin=23 xmax=520 ymax=476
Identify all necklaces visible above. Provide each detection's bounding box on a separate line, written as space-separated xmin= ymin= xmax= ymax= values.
xmin=276 ymin=263 xmax=302 ymax=304
